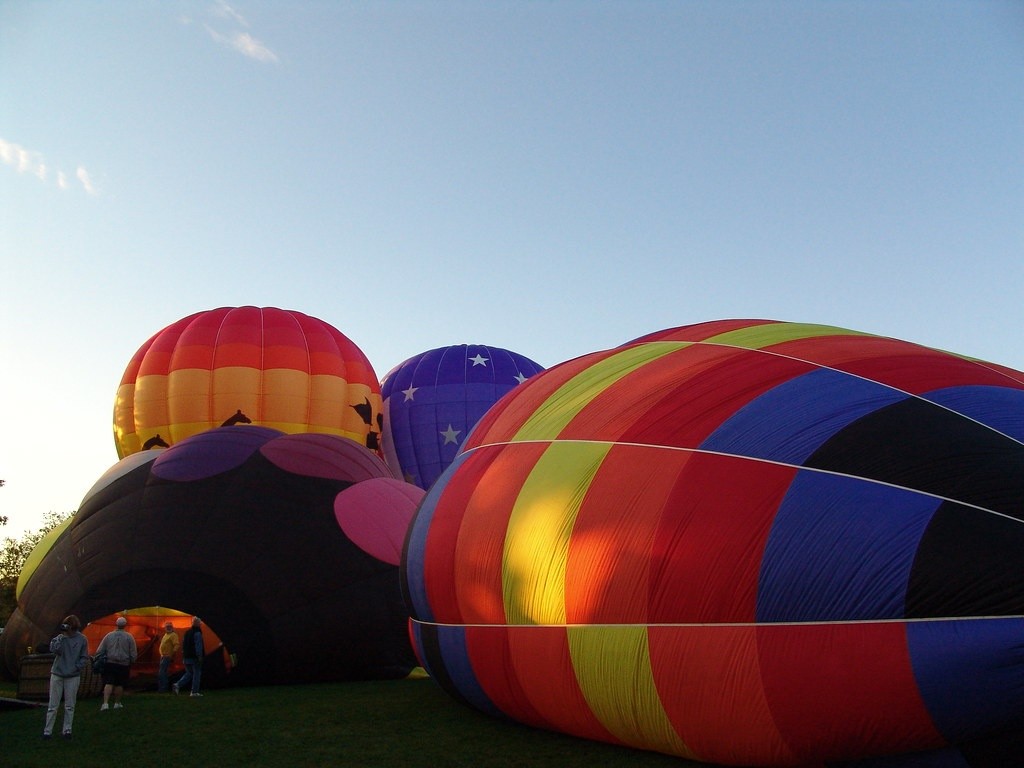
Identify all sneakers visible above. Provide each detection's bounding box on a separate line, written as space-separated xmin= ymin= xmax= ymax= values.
xmin=101 ymin=704 xmax=109 ymax=711
xmin=114 ymin=702 xmax=123 ymax=709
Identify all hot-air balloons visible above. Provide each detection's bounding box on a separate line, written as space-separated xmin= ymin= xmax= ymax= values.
xmin=378 ymin=345 xmax=549 ymax=492
xmin=1 ymin=424 xmax=425 ymax=702
xmin=406 ymin=320 xmax=1024 ymax=768
xmin=112 ymin=304 xmax=384 ymax=461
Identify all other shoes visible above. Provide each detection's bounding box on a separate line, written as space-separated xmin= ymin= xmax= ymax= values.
xmin=190 ymin=692 xmax=203 ymax=697
xmin=64 ymin=734 xmax=72 ymax=741
xmin=173 ymin=684 xmax=180 ymax=696
xmin=43 ymin=736 xmax=52 ymax=741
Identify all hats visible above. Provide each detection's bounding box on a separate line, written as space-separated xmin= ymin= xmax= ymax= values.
xmin=116 ymin=617 xmax=126 ymax=626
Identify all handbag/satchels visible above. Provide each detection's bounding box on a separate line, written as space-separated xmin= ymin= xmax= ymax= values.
xmin=90 ymin=652 xmax=107 ymax=674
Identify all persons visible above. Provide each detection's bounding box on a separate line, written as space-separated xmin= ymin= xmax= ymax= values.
xmin=158 ymin=623 xmax=179 ymax=693
xmin=172 ymin=616 xmax=204 ymax=698
xmin=42 ymin=615 xmax=88 ymax=741
xmin=96 ymin=617 xmax=137 ymax=711
